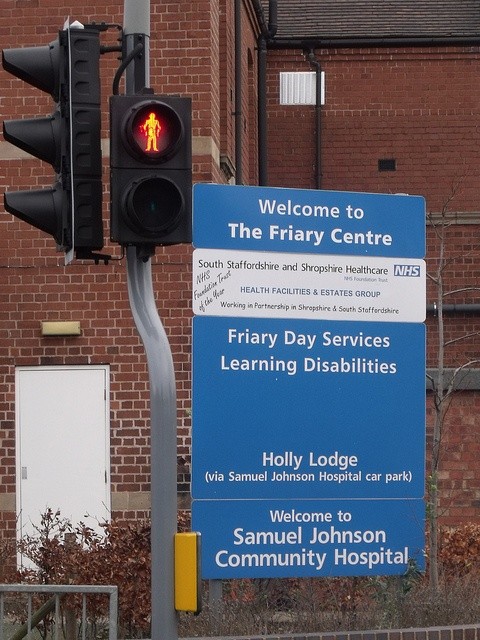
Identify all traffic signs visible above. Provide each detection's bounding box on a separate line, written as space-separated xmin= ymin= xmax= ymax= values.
xmin=196 ymin=181 xmax=434 ymax=584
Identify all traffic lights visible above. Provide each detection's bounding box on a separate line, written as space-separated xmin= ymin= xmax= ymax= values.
xmin=1 ymin=16 xmax=104 ymax=266
xmin=107 ymin=93 xmax=193 ymax=246
